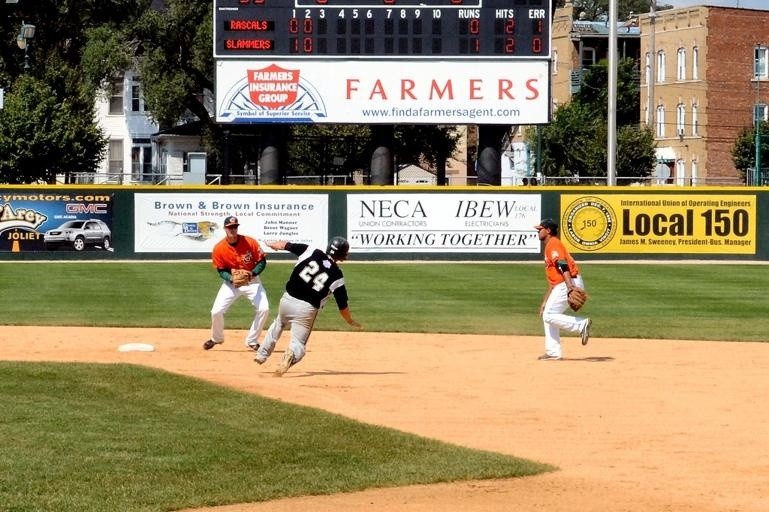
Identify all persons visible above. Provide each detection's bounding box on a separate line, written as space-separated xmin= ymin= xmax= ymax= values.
xmin=533 ymin=218 xmax=592 ymax=360
xmin=254 ymin=236 xmax=362 ymax=377
xmin=202 ymin=216 xmax=270 ymax=351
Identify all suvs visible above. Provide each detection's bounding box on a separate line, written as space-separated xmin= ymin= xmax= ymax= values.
xmin=43 ymin=218 xmax=113 ymax=251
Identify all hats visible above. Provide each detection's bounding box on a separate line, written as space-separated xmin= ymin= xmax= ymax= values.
xmin=535 ymin=219 xmax=558 ymax=230
xmin=224 ymin=217 xmax=240 ymax=227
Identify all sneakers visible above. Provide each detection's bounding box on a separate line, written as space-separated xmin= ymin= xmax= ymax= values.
xmin=539 ymin=353 xmax=560 ymax=360
xmin=581 ymin=318 xmax=591 ymax=344
xmin=202 ymin=339 xmax=218 ymax=351
xmin=274 ymin=350 xmax=296 ymax=375
xmin=246 ymin=342 xmax=259 ymax=350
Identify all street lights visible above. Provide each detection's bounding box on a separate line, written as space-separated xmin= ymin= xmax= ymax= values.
xmin=646 ymin=11 xmax=665 ymax=124
xmin=16 ymin=19 xmax=37 ymax=76
xmin=754 ymin=42 xmax=763 ymax=186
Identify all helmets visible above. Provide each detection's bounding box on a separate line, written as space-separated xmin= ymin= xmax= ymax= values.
xmin=327 ymin=237 xmax=348 ymax=261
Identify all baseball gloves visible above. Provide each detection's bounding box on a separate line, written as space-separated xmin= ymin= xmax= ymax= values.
xmin=566 ymin=286 xmax=587 ymax=310
xmin=230 ymin=267 xmax=251 ymax=288
